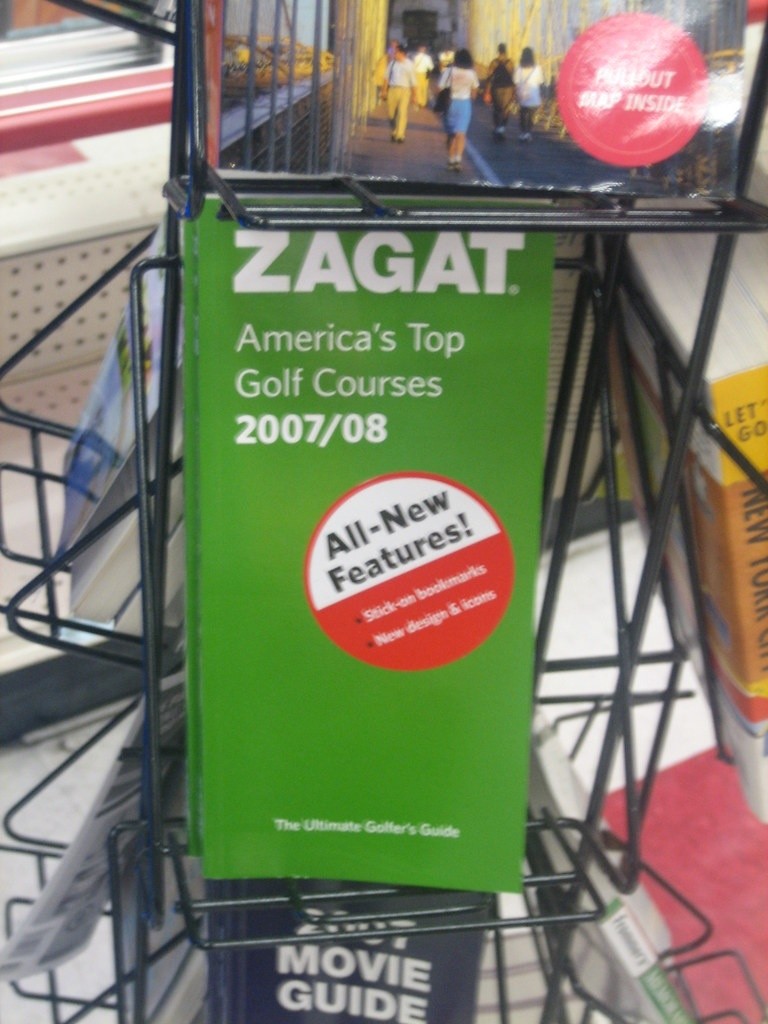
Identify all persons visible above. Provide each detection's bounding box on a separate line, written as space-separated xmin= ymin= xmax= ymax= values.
xmin=381 ymin=39 xmax=544 ymax=173
